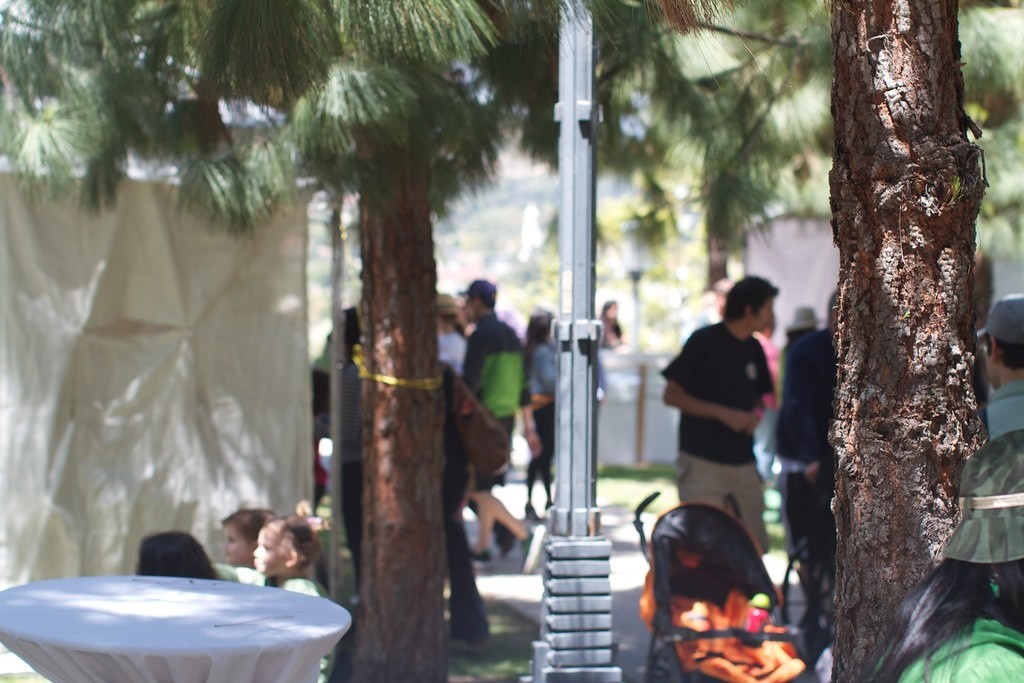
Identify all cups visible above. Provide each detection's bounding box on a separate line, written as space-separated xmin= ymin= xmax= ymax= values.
xmin=743 ymin=593 xmax=771 ymax=649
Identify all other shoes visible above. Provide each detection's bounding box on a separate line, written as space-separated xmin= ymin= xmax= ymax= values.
xmin=521 ymin=524 xmax=546 ymax=576
xmin=471 ymin=548 xmax=496 ymax=572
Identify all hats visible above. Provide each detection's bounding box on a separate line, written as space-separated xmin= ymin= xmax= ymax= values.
xmin=457 ymin=280 xmax=498 ymax=302
xmin=785 ymin=305 xmax=823 ymax=332
xmin=977 ymin=292 xmax=1024 ymax=345
xmin=436 ymin=293 xmax=462 ymax=317
xmin=942 ymin=429 xmax=1023 ymax=562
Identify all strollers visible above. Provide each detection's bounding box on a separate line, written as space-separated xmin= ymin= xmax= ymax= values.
xmin=632 ymin=492 xmax=813 ymax=682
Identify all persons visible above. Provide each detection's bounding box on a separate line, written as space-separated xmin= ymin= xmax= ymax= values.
xmin=251 ymin=516 xmax=340 ymax=683
xmin=658 ymin=277 xmax=780 ymax=569
xmin=773 ymin=286 xmax=851 ymax=655
xmin=673 ymin=281 xmax=822 ymax=485
xmin=971 ymin=293 xmax=1023 ymax=436
xmin=135 ymin=530 xmax=218 ymax=578
xmin=846 ymin=425 xmax=1024 ymax=683
xmin=314 ymin=277 xmax=632 ymax=640
xmin=210 ymin=507 xmax=274 ymax=585
